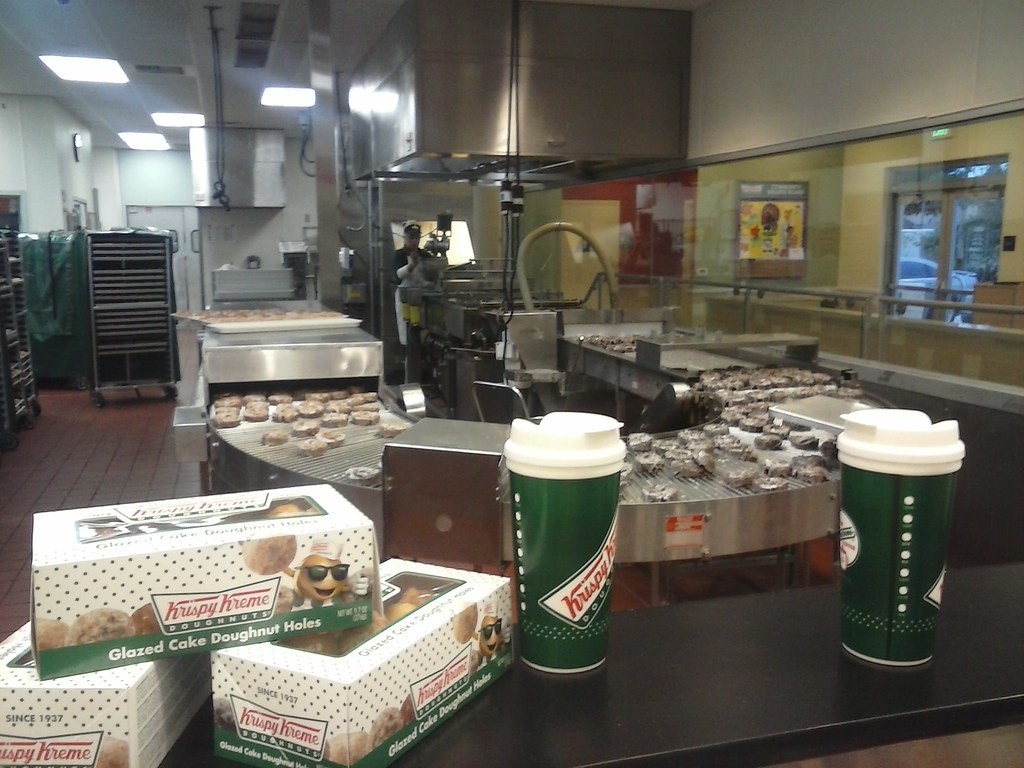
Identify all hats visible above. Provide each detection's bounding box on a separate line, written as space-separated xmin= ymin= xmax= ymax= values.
xmin=404 ymin=224 xmax=421 ymax=236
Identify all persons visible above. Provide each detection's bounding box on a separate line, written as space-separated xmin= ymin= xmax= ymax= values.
xmin=391 ymin=221 xmax=434 ymax=345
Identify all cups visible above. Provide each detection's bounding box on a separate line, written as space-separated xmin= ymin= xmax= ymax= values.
xmin=502 ymin=411 xmax=628 ymax=674
xmin=836 ymin=408 xmax=968 ymax=666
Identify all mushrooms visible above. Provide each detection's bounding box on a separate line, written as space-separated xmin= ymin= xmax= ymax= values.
xmin=452 ymin=603 xmax=480 ymax=643
xmin=241 ymin=535 xmax=298 ymax=578
xmin=35 ymin=602 xmax=162 ymax=652
xmin=326 ymin=692 xmax=416 ymax=767
xmin=91 ymin=736 xmax=130 ymax=768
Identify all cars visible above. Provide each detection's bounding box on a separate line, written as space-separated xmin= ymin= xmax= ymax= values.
xmin=895 ymin=256 xmax=979 ymax=320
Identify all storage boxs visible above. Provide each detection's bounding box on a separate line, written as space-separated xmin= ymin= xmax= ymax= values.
xmin=210 ymin=557 xmax=513 ymax=768
xmin=0 ymin=619 xmax=213 ymax=768
xmin=27 ymin=483 xmax=383 ymax=683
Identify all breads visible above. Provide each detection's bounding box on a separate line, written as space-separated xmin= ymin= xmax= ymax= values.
xmin=622 ymin=366 xmax=840 ymax=503
xmin=213 ymin=385 xmax=406 ymax=457
